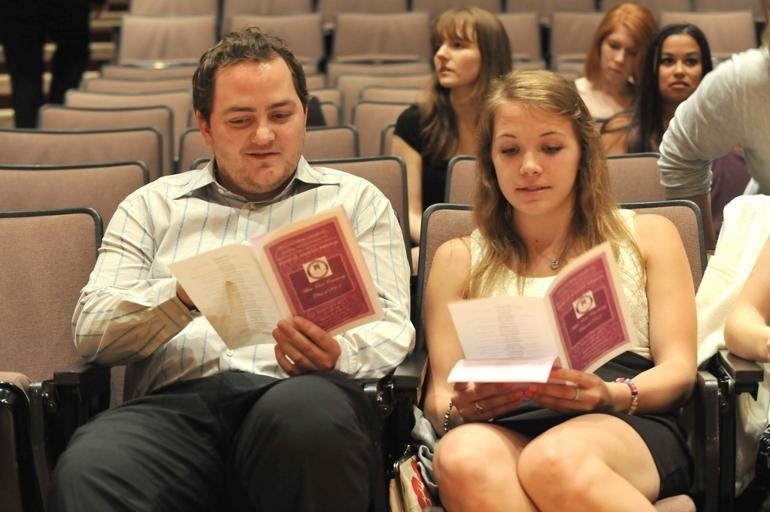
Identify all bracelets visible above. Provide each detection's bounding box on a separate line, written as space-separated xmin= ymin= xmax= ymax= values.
xmin=617 ymin=376 xmax=639 ymax=417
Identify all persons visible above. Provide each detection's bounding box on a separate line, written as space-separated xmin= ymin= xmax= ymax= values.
xmin=420 ymin=68 xmax=699 ymax=512
xmin=1 ymin=3 xmax=89 ymax=129
xmin=657 ymin=0 xmax=770 ymax=248
xmin=48 ymin=26 xmax=418 ymax=512
xmin=574 ymin=0 xmax=713 ymax=157
xmin=391 ymin=7 xmax=512 ymax=240
xmin=723 ymin=234 xmax=770 ymax=476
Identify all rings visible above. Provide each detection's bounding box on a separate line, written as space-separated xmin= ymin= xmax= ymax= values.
xmin=474 ymin=401 xmax=484 ymax=413
xmin=574 ymin=386 xmax=582 ymax=402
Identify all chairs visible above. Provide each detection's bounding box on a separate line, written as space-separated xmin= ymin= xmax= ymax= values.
xmin=0 ymin=2 xmax=770 ymax=507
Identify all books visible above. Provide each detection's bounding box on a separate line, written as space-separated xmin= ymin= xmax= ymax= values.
xmin=443 ymin=240 xmax=633 ymax=401
xmin=168 ymin=204 xmax=384 ymax=351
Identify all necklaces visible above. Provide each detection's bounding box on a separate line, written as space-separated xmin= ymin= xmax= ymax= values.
xmin=530 ymin=240 xmax=573 ymax=270
